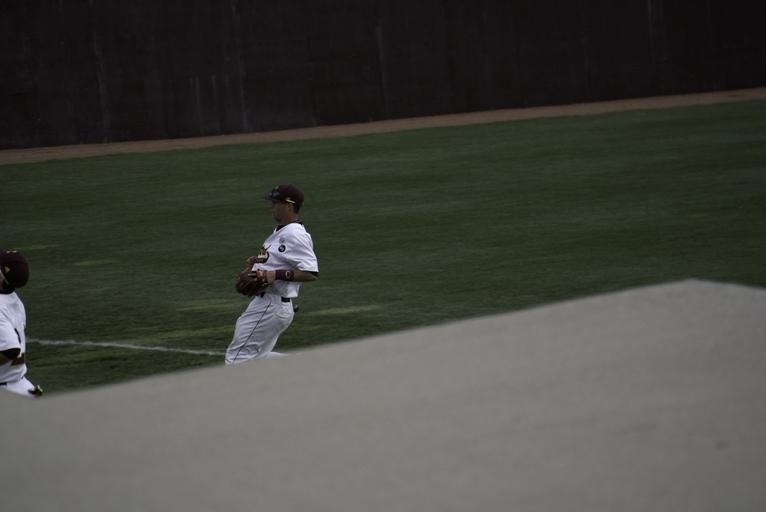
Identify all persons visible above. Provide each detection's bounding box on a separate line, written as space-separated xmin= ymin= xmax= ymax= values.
xmin=0 ymin=248 xmax=43 ymax=399
xmin=223 ymin=183 xmax=321 ymax=366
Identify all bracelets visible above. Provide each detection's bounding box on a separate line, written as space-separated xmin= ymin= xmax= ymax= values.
xmin=274 ymin=269 xmax=295 ymax=281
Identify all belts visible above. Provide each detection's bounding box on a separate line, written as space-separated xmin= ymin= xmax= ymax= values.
xmin=260 ymin=292 xmax=292 ymax=303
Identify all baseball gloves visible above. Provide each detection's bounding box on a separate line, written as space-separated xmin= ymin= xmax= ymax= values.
xmin=235 ymin=270 xmax=269 ymax=299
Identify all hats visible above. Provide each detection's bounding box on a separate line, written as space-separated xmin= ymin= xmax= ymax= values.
xmin=261 ymin=182 xmax=304 ymax=209
xmin=0 ymin=248 xmax=31 ymax=289
